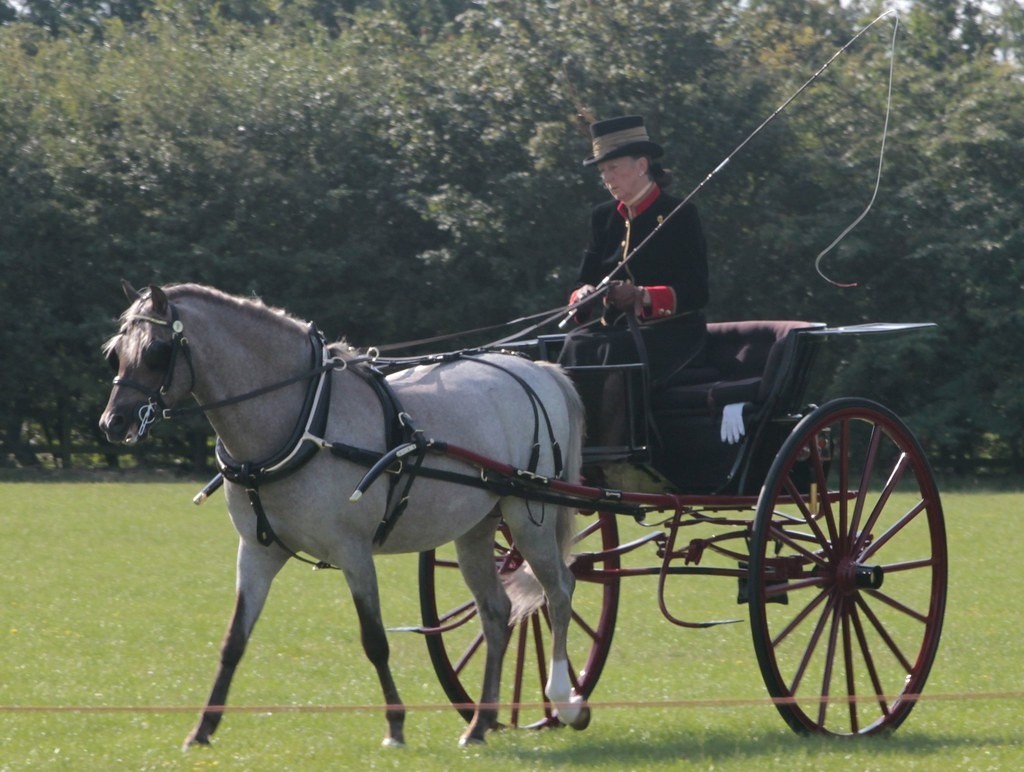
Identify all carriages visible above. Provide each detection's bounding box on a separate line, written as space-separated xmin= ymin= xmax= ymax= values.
xmin=98 ymin=279 xmax=949 ymax=747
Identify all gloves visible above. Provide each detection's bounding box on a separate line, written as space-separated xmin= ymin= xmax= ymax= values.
xmin=720 ymin=402 xmax=747 ymax=444
xmin=603 ymin=280 xmax=643 ymax=310
xmin=569 ymin=285 xmax=596 ymax=314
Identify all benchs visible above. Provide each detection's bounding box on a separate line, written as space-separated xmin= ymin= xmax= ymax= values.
xmin=638 ymin=319 xmax=825 ymax=417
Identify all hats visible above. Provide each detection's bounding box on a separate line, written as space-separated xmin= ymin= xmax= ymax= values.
xmin=582 ymin=116 xmax=665 ymax=166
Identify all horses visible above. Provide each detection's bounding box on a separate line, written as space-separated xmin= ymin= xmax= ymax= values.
xmin=98 ymin=276 xmax=592 ymax=751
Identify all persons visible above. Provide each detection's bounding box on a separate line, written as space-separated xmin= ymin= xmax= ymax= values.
xmin=557 ymin=116 xmax=710 ymax=487
